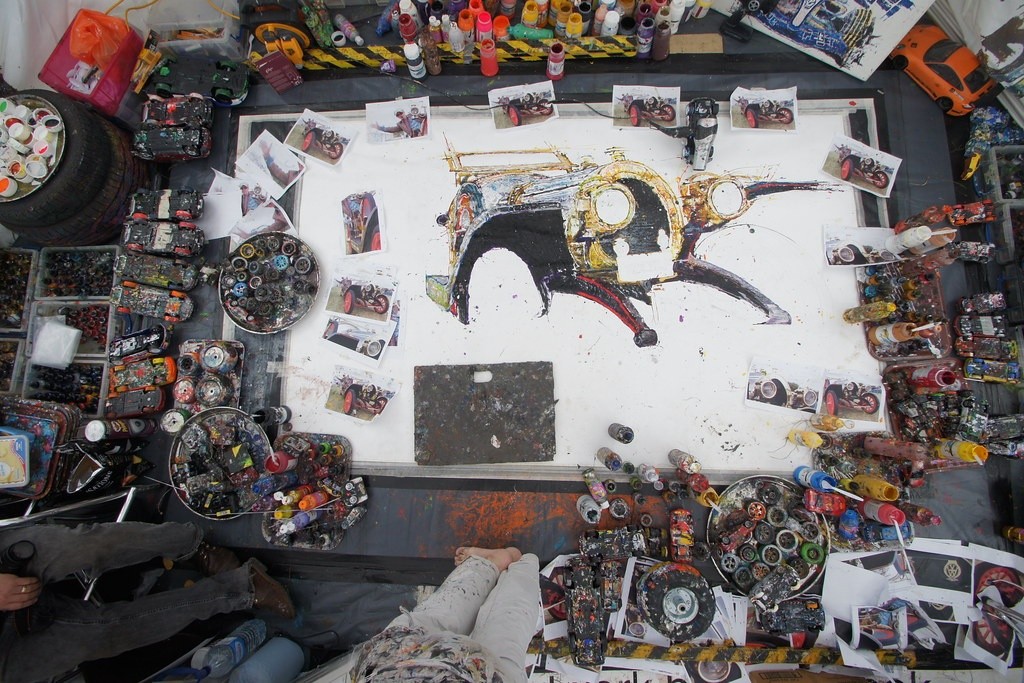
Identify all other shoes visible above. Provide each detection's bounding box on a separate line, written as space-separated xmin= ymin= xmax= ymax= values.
xmin=191 ymin=537 xmax=239 ymax=576
xmin=245 ymin=557 xmax=295 ymax=620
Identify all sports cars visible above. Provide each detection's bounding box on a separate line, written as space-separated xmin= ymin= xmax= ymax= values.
xmin=895 ymin=205 xmax=949 ymax=234
xmin=563 ymin=509 xmax=695 ymax=668
xmin=954 ymin=291 xmax=1020 ymax=387
xmin=947 ymin=199 xmax=997 ymax=227
xmin=649 ymin=97 xmax=721 ymax=171
xmin=104 ymin=185 xmax=206 ymax=420
xmin=955 ymin=394 xmax=1024 ymax=461
xmin=131 ymin=55 xmax=250 ymax=163
xmin=949 ymin=241 xmax=997 ymax=264
xmin=888 ymin=24 xmax=1004 ymax=120
xmin=716 ymin=514 xmax=758 ymax=554
xmin=751 ymin=564 xmax=828 ymax=636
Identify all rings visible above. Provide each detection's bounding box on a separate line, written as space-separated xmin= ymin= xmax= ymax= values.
xmin=21 ymin=586 xmax=29 ymax=593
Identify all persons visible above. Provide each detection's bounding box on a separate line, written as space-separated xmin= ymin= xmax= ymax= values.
xmin=0 ymin=520 xmax=301 ymax=682
xmin=350 ymin=547 xmax=546 ymax=683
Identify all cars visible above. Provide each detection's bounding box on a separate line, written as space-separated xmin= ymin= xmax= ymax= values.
xmin=506 ymin=92 xmax=554 ymax=127
xmin=344 ymin=282 xmax=389 ymax=316
xmin=824 ymin=379 xmax=880 ymax=416
xmin=738 ymin=96 xmax=795 ymax=129
xmin=627 ymin=96 xmax=676 ymax=127
xmin=343 ymin=380 xmax=388 ymax=418
xmin=300 ymin=119 xmax=344 ymax=160
xmin=839 ymin=153 xmax=889 ymax=190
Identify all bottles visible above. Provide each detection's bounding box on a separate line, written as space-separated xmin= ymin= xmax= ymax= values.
xmin=2 ymin=0 xmax=1024 ymax=683
